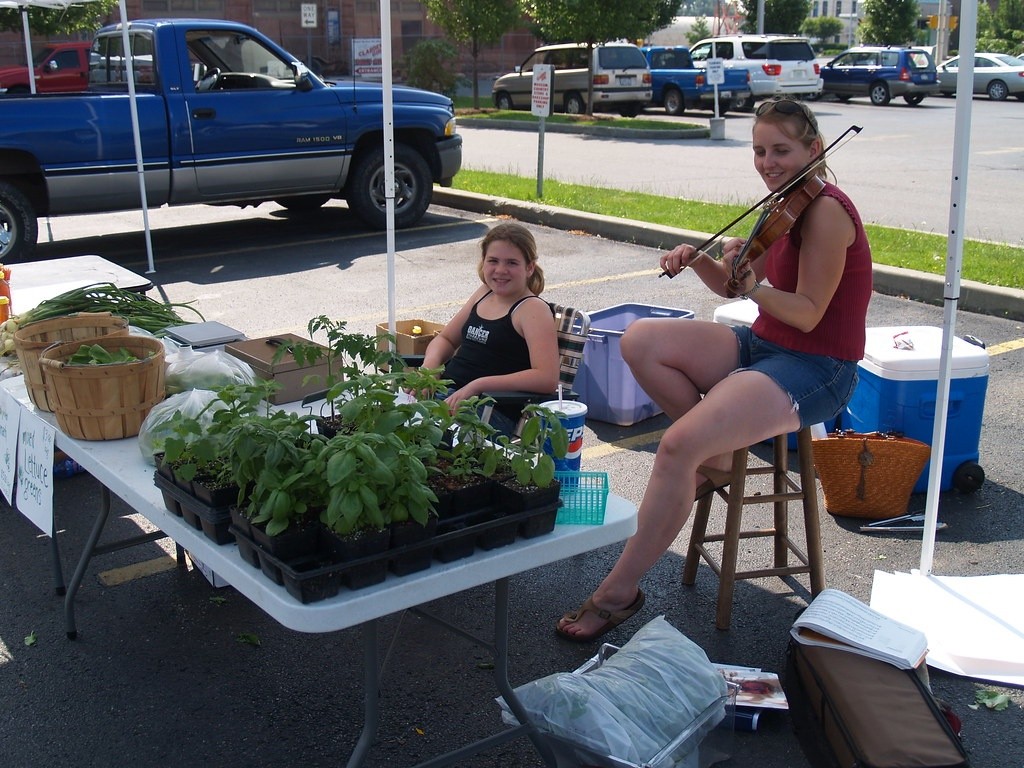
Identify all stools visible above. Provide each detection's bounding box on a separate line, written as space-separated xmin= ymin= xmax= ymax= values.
xmin=681 ymin=426 xmax=825 ymax=631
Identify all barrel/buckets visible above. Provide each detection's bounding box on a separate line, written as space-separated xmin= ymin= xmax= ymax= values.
xmin=38 ymin=334 xmax=166 ymax=440
xmin=12 ymin=311 xmax=131 ymax=411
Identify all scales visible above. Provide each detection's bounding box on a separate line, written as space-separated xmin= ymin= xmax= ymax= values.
xmin=164 ymin=321 xmax=250 ymax=355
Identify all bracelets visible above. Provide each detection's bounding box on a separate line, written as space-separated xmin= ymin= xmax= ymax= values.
xmin=740 ymin=279 xmax=762 ymax=300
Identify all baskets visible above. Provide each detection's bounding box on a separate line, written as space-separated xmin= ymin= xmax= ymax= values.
xmin=552 ymin=471 xmax=609 ymax=526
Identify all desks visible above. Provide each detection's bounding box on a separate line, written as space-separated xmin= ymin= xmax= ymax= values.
xmin=0 ymin=255 xmax=155 ymax=317
xmin=0 ymin=366 xmax=637 ymax=768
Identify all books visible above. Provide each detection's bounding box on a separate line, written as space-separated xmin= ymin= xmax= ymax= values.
xmin=790 ymin=588 xmax=930 ymax=671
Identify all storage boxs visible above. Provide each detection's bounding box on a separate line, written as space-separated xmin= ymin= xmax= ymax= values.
xmin=501 ymin=643 xmax=740 ymax=768
xmin=570 ymin=303 xmax=694 ymax=427
xmin=714 ymin=299 xmax=839 ymax=452
xmin=164 ymin=321 xmax=243 ymax=354
xmin=839 ymin=324 xmax=991 ymax=496
xmin=554 ymin=471 xmax=610 ymax=526
xmin=227 ymin=500 xmax=564 ymax=604
xmin=152 ymin=471 xmax=237 ymax=546
xmin=375 ymin=319 xmax=447 ymax=374
xmin=224 ymin=334 xmax=344 ymax=408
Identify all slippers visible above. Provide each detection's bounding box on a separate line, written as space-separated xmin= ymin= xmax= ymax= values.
xmin=695 ymin=464 xmax=734 ymax=501
xmin=555 ymin=585 xmax=646 ymax=644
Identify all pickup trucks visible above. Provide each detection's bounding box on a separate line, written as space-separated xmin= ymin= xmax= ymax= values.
xmin=639 ymin=46 xmax=751 ymax=115
xmin=0 ymin=17 xmax=464 ymax=267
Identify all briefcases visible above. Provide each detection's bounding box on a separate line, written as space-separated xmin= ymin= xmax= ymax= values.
xmin=224 ymin=333 xmax=344 ymax=407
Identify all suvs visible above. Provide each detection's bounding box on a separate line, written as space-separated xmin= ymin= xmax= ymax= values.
xmin=820 ymin=45 xmax=941 ymax=107
xmin=688 ymin=34 xmax=824 ymax=113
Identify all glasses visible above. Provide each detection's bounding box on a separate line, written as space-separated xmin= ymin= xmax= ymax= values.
xmin=756 ymin=100 xmax=817 ymax=139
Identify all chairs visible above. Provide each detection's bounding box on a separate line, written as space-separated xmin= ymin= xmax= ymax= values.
xmin=706 ymin=46 xmax=712 ymax=58
xmin=388 ymin=302 xmax=591 ymax=450
xmin=550 ymin=56 xmax=564 ymax=69
xmin=575 ymin=57 xmax=588 ymax=67
xmin=717 ymin=47 xmax=729 ymax=58
xmin=664 ymin=57 xmax=676 ymax=67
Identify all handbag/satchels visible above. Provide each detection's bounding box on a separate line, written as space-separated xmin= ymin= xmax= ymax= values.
xmin=496 ymin=614 xmax=728 ymax=768
xmin=137 ymin=388 xmax=251 ymax=469
xmin=810 ymin=427 xmax=931 ymax=518
xmin=125 ymin=320 xmax=259 ymax=396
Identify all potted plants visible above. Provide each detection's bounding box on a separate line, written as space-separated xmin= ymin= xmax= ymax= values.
xmin=150 ymin=314 xmax=569 ymax=570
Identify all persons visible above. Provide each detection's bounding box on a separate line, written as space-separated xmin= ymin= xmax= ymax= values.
xmin=403 ymin=222 xmax=561 ymax=447
xmin=556 ymin=99 xmax=873 ymax=639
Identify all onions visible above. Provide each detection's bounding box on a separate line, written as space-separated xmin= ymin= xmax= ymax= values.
xmin=0 ymin=319 xmax=18 ymax=355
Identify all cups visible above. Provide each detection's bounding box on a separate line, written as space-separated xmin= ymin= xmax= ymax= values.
xmin=537 ymin=400 xmax=588 ymax=495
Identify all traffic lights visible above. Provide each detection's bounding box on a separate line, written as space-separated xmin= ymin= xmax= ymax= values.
xmin=949 ymin=17 xmax=958 ymax=29
xmin=926 ymin=15 xmax=939 ymax=28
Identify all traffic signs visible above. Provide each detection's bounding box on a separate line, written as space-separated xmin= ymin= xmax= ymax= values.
xmin=301 ymin=4 xmax=317 ymax=28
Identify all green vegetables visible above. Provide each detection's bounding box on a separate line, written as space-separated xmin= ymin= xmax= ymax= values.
xmin=62 ymin=344 xmax=156 ymax=365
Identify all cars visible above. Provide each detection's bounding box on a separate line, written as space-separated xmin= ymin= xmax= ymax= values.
xmin=935 ymin=53 xmax=1024 ymax=101
xmin=1008 ymin=54 xmax=1024 ymax=66
xmin=0 ymin=42 xmax=93 ymax=94
xmin=492 ymin=41 xmax=653 ymax=118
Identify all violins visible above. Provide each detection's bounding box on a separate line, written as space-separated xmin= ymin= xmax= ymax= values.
xmin=721 ymin=172 xmax=827 ymax=299
